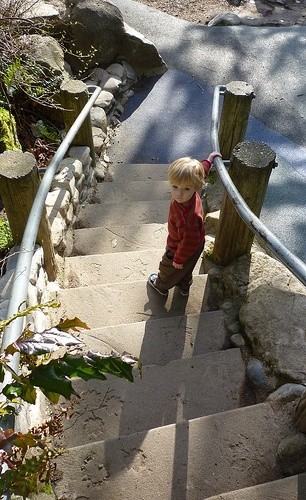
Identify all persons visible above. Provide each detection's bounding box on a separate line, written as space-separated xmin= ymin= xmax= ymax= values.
xmin=148 ymin=151 xmax=222 ymax=296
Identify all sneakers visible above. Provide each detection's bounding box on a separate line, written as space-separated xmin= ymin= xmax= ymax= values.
xmin=176 ymin=286 xmax=189 ymax=296
xmin=149 ymin=273 xmax=168 ymax=295
